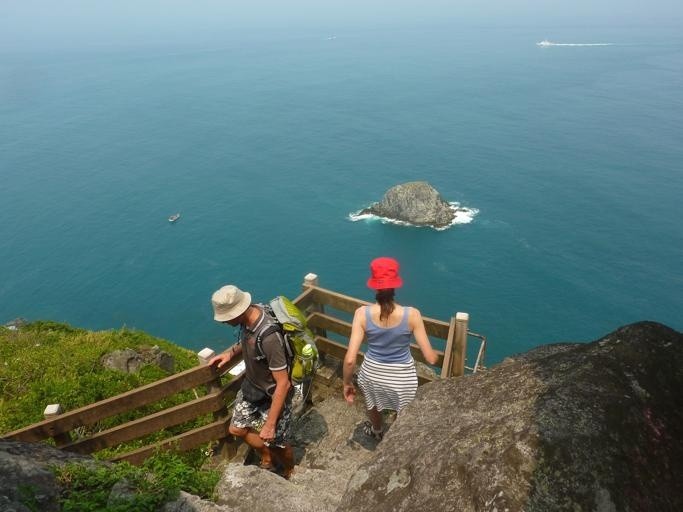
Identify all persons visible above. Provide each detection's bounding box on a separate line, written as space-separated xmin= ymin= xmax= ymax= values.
xmin=343 ymin=257 xmax=439 ymax=442
xmin=207 ymin=283 xmax=319 ymax=481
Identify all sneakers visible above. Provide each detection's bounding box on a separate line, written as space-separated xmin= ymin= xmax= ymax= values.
xmin=282 ymin=463 xmax=293 ymax=480
xmin=259 ymin=457 xmax=273 ymax=471
xmin=364 ymin=427 xmax=382 ymax=441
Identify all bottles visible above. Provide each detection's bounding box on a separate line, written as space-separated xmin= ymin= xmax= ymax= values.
xmin=300 ymin=344 xmax=312 ymax=374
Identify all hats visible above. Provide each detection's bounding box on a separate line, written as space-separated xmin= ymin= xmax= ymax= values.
xmin=210 ymin=284 xmax=251 ymax=323
xmin=366 ymin=257 xmax=403 ymax=290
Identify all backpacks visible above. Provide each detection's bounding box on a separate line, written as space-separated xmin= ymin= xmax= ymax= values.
xmin=255 ymin=294 xmax=319 ymax=406
